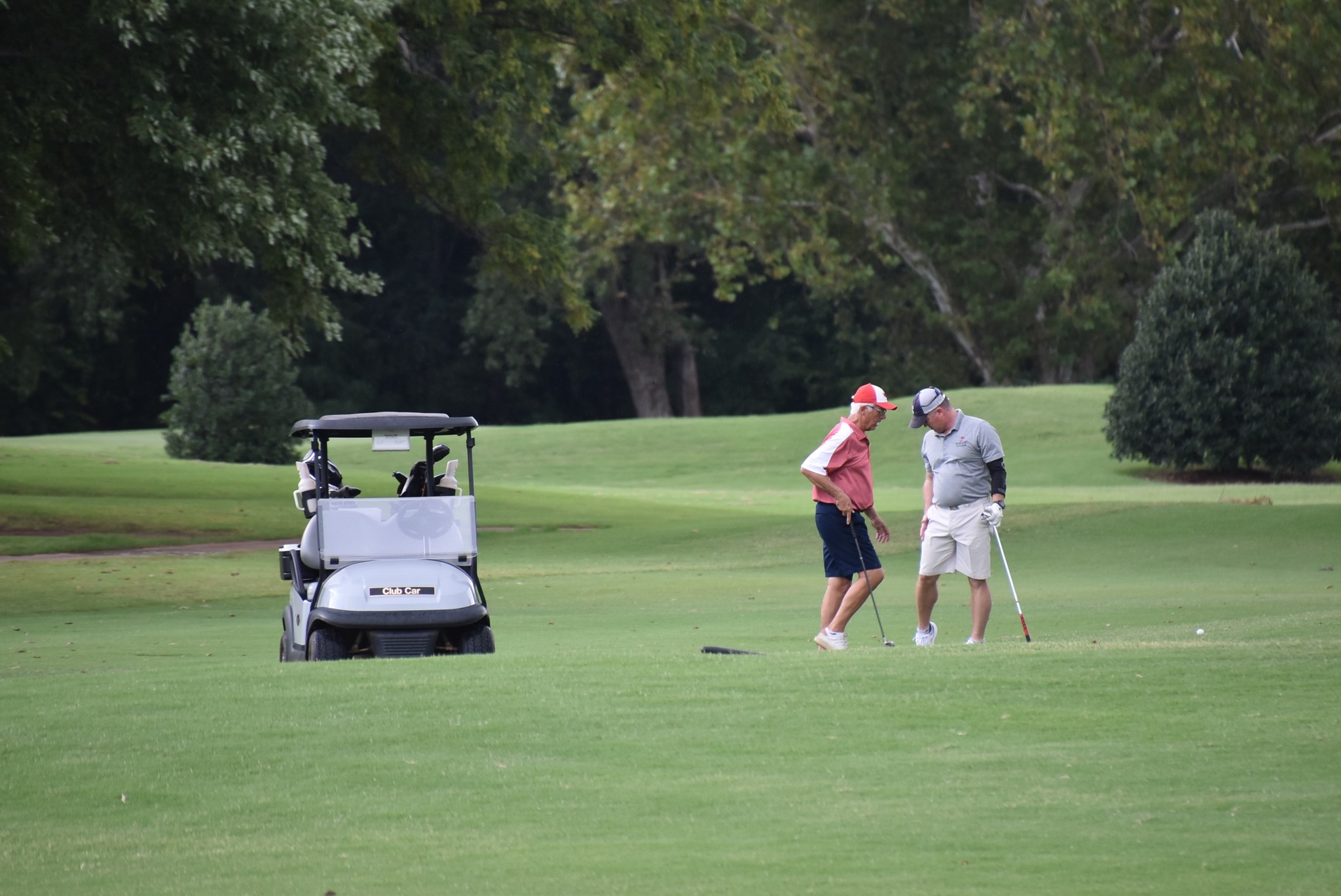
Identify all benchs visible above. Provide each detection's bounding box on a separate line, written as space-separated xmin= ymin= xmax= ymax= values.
xmin=299 ymin=507 xmax=464 ymax=568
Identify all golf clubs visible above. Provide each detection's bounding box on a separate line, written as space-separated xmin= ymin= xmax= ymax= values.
xmin=980 ymin=512 xmax=1031 ymax=643
xmin=850 ymin=516 xmax=896 ymax=646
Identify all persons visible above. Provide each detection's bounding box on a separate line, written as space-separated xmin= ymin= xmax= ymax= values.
xmin=908 ymin=386 xmax=1007 ymax=645
xmin=800 ymin=383 xmax=898 ymax=650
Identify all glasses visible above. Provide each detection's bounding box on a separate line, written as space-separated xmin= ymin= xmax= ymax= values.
xmin=866 ymin=405 xmax=887 ymax=415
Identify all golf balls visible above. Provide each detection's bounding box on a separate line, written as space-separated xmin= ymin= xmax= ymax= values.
xmin=1197 ymin=629 xmax=1204 ymax=635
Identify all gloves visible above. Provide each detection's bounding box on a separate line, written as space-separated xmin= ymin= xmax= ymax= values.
xmin=985 ymin=503 xmax=1003 ymax=528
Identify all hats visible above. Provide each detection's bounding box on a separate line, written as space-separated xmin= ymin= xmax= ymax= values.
xmin=908 ymin=386 xmax=946 ymax=429
xmin=852 ymin=382 xmax=898 ymax=410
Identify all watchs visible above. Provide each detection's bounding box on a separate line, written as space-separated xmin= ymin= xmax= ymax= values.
xmin=993 ymin=500 xmax=1007 ymax=509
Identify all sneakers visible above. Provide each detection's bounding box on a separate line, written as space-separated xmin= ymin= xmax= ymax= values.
xmin=813 ymin=627 xmax=849 ymax=651
xmin=912 ymin=621 xmax=938 ymax=646
xmin=965 ymin=637 xmax=984 ymax=644
xmin=818 ymin=646 xmax=825 ymax=650
